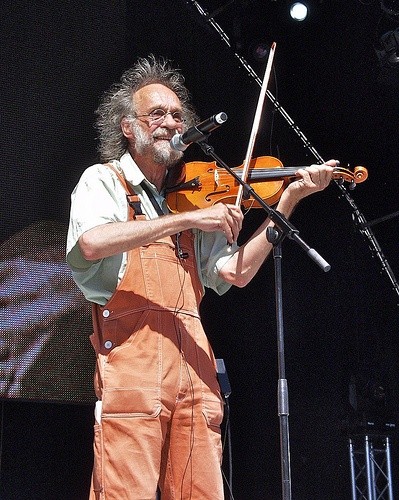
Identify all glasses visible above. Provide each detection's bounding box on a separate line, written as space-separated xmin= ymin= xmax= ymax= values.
xmin=133 ymin=108 xmax=184 ymax=125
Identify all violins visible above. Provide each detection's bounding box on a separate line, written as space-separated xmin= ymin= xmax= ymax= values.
xmin=164 ymin=152 xmax=369 ymax=216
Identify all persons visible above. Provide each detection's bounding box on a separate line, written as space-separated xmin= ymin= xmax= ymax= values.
xmin=65 ymin=53 xmax=340 ymax=500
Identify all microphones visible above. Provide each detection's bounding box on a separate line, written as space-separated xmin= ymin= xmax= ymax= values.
xmin=170 ymin=112 xmax=227 ymax=151
